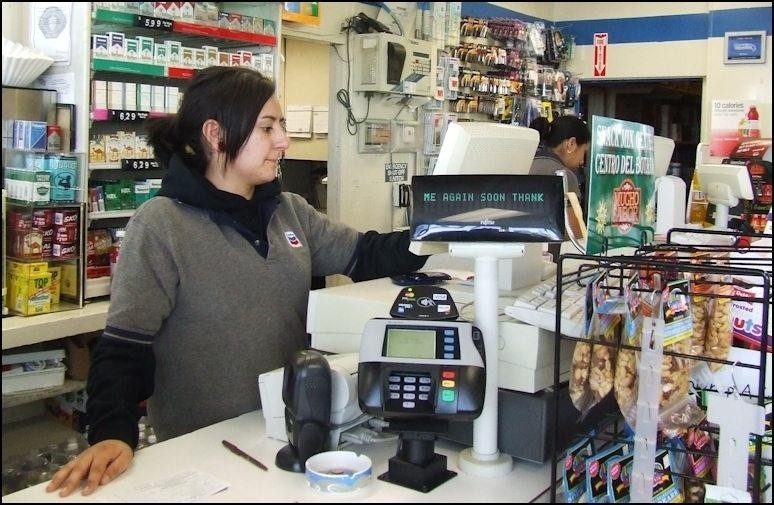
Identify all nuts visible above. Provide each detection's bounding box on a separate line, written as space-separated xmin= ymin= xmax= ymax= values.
xmin=568 ymin=282 xmax=733 ymax=413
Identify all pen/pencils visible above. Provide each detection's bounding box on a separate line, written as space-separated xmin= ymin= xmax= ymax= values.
xmin=222 ymin=439 xmax=268 ymax=472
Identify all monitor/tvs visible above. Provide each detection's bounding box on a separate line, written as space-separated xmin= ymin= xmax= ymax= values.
xmin=694 ymin=162 xmax=753 ymax=207
xmin=407 ymin=123 xmax=540 ymax=256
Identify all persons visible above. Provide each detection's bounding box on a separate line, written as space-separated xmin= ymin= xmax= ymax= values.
xmin=46 ymin=65 xmax=430 ymax=497
xmin=529 ymin=114 xmax=591 ymax=241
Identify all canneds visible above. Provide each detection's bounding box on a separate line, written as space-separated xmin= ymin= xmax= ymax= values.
xmin=47 ymin=126 xmax=61 ymax=150
xmin=8 ymin=208 xmax=79 ymax=259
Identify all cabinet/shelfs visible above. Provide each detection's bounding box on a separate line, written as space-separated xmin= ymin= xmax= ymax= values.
xmin=35 ymin=0 xmax=283 ymax=302
xmin=553 ymin=228 xmax=771 ymax=501
xmin=4 ymin=303 xmax=166 ymax=496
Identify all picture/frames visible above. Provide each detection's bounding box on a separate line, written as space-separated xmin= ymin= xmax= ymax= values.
xmin=358 ymin=117 xmax=391 ymax=154
xmin=725 ymin=29 xmax=767 ymax=64
xmin=391 ymin=120 xmax=421 ymax=153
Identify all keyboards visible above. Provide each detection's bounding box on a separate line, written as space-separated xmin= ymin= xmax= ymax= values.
xmin=508 ymin=269 xmax=622 ymax=337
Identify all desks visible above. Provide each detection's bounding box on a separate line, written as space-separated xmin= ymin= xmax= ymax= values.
xmin=5 ymin=376 xmax=593 ymax=505
